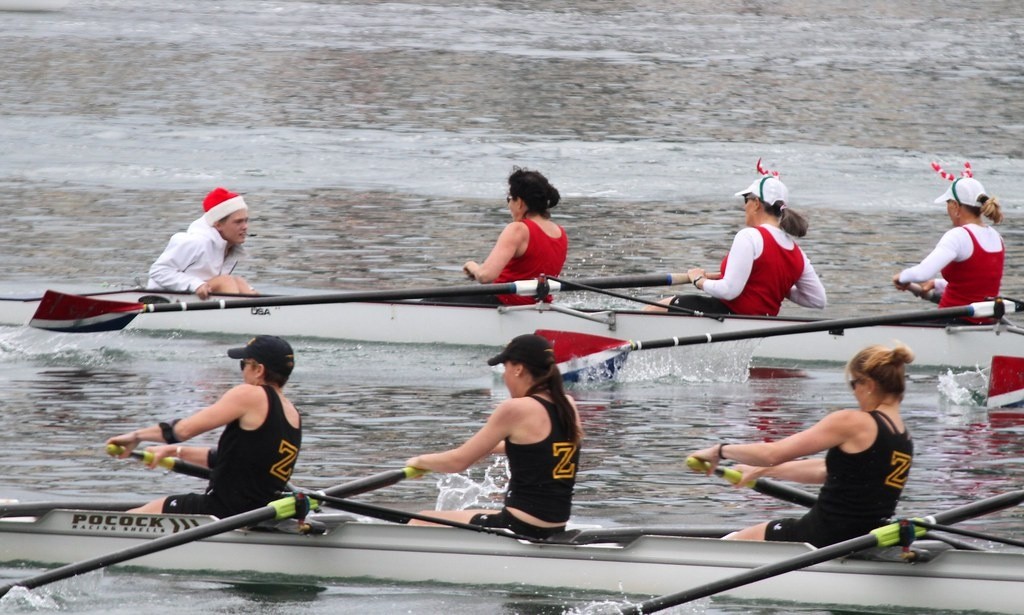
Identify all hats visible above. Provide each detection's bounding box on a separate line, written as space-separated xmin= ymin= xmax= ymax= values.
xmin=227 ymin=335 xmax=295 ymax=375
xmin=931 ymin=177 xmax=986 ymax=207
xmin=734 ymin=177 xmax=789 ymax=208
xmin=202 ymin=187 xmax=247 ymax=227
xmin=487 ymin=334 xmax=554 ymax=367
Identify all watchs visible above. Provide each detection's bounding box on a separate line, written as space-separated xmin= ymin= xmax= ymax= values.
xmin=693 ymin=275 xmax=703 ymax=289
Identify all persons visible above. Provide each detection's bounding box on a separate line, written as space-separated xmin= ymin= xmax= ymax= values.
xmin=405 ymin=334 xmax=583 ymax=535
xmin=418 ymin=164 xmax=567 ymax=308
xmin=894 ymin=178 xmax=1005 ymax=328
xmin=639 ymin=178 xmax=825 ymax=316
xmin=146 ymin=188 xmax=259 ymax=301
xmin=104 ymin=334 xmax=303 ymax=519
xmin=689 ymin=344 xmax=914 ymax=551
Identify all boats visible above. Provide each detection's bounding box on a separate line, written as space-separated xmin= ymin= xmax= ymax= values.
xmin=1 ymin=481 xmax=1024 ymax=615
xmin=0 ymin=283 xmax=1024 ymax=371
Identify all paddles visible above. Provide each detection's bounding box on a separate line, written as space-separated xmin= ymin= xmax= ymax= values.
xmin=107 ymin=441 xmax=411 ymax=523
xmin=0 ymin=465 xmax=425 ymax=597
xmin=686 ymin=457 xmax=986 ymax=549
xmin=30 ymin=272 xmax=722 ymax=334
xmin=0 ymin=500 xmax=148 ymax=518
xmin=895 ymin=278 xmax=946 ymax=305
xmin=608 ymin=488 xmax=1023 ymax=615
xmin=534 ymin=298 xmax=1022 ymax=385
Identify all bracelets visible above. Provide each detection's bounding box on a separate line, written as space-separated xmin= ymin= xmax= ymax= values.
xmin=176 ymin=446 xmax=182 ymax=458
xmin=719 ymin=443 xmax=729 ymax=460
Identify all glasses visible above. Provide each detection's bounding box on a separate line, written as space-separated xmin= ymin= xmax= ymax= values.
xmin=238 ymin=360 xmax=255 ymax=372
xmin=849 ymin=379 xmax=863 ymax=390
xmin=744 ymin=195 xmax=757 ymax=204
xmin=505 ymin=195 xmax=514 ymax=203
